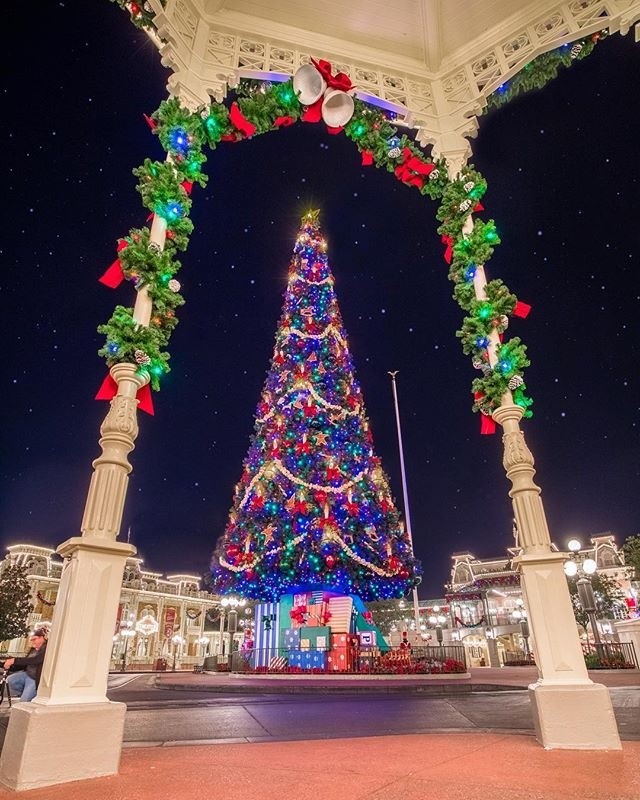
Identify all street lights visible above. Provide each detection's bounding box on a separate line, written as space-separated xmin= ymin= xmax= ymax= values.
xmin=119 ymin=613 xmax=134 ymax=672
xmin=220 ymin=591 xmax=246 ymax=676
xmin=199 ymin=637 xmax=210 ymax=674
xmin=563 ymin=539 xmax=614 ymax=670
xmin=511 ymin=598 xmax=534 ymax=666
xmin=171 ymin=635 xmax=182 ymax=673
xmin=427 ymin=605 xmax=447 ymax=674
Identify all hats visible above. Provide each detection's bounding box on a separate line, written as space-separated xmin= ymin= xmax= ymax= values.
xmin=33 ymin=630 xmax=45 ymax=636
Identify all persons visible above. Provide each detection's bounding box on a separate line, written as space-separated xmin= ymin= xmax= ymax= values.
xmin=4 ymin=625 xmax=49 ymax=703
